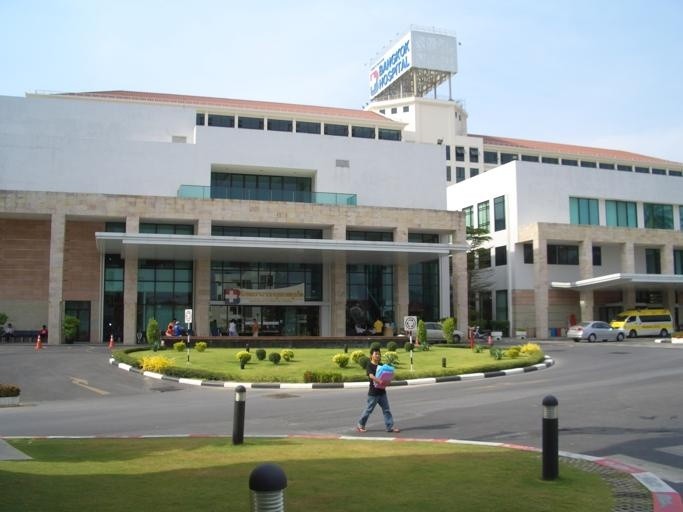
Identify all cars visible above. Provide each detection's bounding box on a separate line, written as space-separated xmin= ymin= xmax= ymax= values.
xmin=566 ymin=320 xmax=626 ymax=343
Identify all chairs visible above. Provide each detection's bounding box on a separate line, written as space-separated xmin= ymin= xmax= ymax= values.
xmin=12 ymin=331 xmax=47 ymax=343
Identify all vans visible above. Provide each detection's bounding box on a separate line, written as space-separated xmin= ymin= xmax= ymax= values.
xmin=606 ymin=307 xmax=675 ymax=338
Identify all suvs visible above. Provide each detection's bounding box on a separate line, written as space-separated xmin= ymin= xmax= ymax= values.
xmin=406 ymin=322 xmax=464 ymax=344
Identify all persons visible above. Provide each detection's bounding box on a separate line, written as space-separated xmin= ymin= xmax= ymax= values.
xmin=2 ymin=323 xmax=16 ymax=343
xmin=164 ymin=318 xmax=184 ymax=336
xmin=38 ymin=325 xmax=48 ymax=341
xmin=356 ymin=347 xmax=400 ymax=432
xmin=228 ymin=318 xmax=239 ymax=336
xmin=373 ymin=317 xmax=384 ymax=336
xmin=251 ymin=318 xmax=259 ymax=336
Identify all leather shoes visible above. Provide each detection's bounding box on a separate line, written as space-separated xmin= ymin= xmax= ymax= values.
xmin=357 ymin=427 xmax=366 ymax=432
xmin=390 ymin=427 xmax=400 ymax=432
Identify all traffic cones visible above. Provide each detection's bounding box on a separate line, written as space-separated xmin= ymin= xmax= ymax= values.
xmin=488 ymin=335 xmax=493 ymax=344
xmin=106 ymin=334 xmax=116 ymax=349
xmin=33 ymin=335 xmax=44 ymax=349
